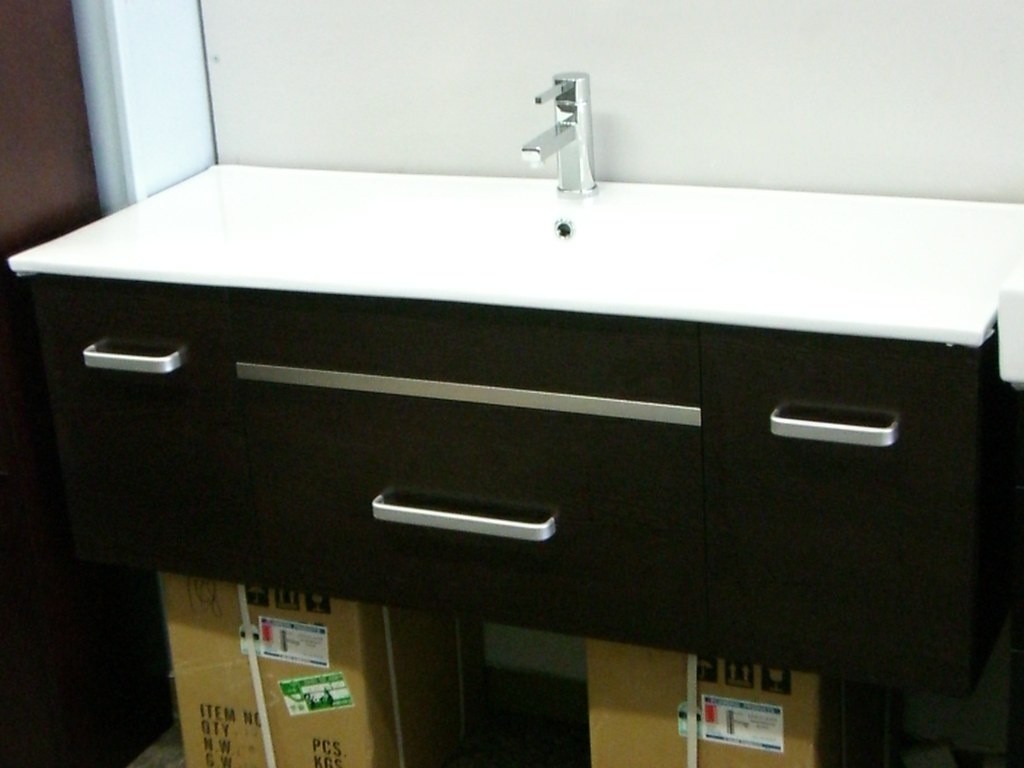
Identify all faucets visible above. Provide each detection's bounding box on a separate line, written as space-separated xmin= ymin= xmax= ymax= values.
xmin=520 ymin=72 xmax=600 ymax=200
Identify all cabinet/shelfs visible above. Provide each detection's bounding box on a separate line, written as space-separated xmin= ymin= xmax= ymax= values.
xmin=0 ymin=272 xmax=1024 ymax=768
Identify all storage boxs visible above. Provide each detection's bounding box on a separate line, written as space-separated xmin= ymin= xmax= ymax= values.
xmin=586 ymin=638 xmax=828 ymax=768
xmin=157 ymin=569 xmax=441 ymax=768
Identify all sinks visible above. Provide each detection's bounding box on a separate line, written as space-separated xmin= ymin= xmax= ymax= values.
xmin=6 ymin=161 xmax=1024 ymax=347
